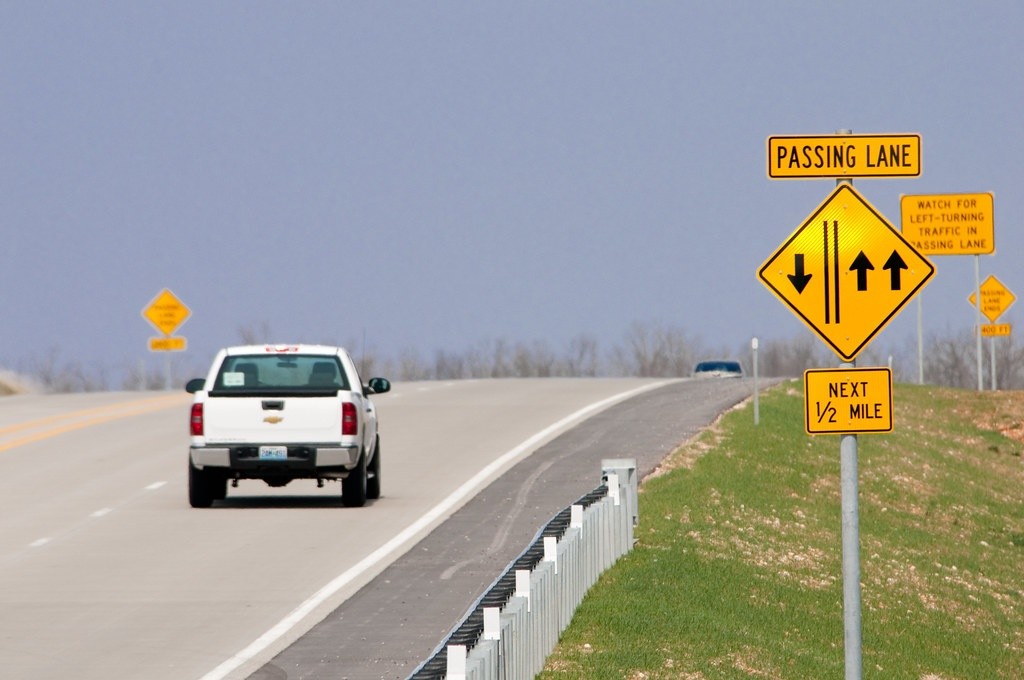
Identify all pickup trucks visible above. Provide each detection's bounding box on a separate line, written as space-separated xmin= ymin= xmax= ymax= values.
xmin=183 ymin=342 xmax=392 ymax=507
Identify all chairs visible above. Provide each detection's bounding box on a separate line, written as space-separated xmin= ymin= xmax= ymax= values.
xmin=308 ymin=374 xmax=334 ymax=387
xmin=244 ymin=375 xmax=258 ymax=386
xmin=233 ymin=364 xmax=259 ymax=374
xmin=313 ymin=362 xmax=336 ymax=377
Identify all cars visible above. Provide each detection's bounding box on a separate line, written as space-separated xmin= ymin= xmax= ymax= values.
xmin=691 ymin=360 xmax=744 ymax=380
